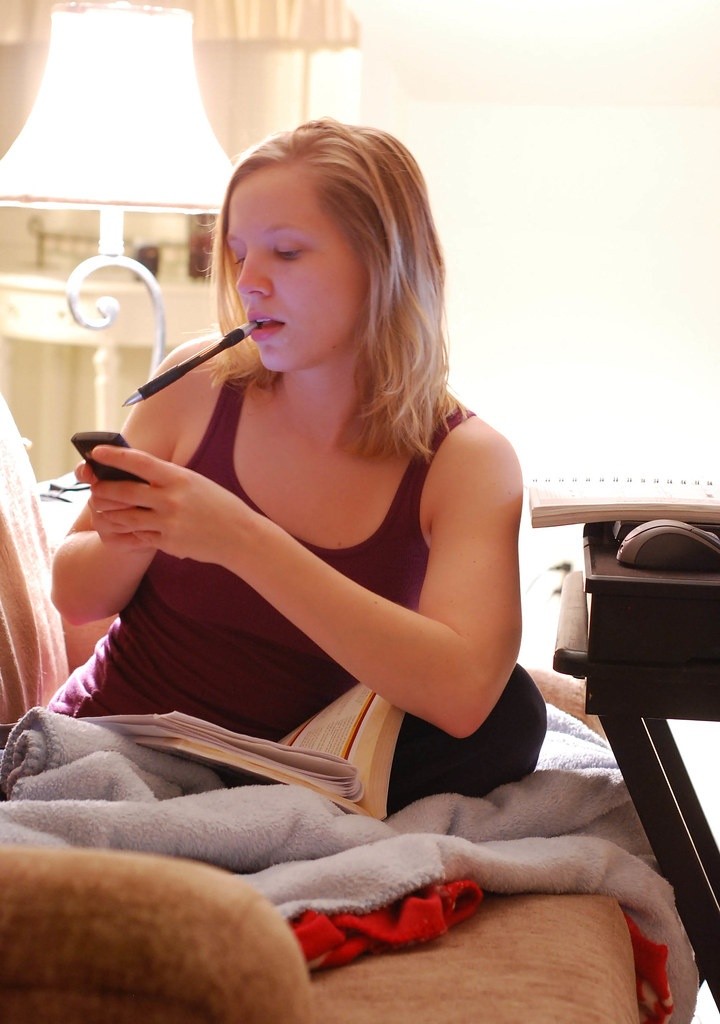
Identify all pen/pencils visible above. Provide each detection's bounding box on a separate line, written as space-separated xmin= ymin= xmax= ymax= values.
xmin=122 ymin=320 xmax=265 ymax=407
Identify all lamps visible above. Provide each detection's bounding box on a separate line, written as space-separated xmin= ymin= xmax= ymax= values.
xmin=0 ymin=1 xmax=235 ymax=432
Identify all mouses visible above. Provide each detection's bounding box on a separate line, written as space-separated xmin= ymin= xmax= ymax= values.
xmin=616 ymin=520 xmax=720 ymax=574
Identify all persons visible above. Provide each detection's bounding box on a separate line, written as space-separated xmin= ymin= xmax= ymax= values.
xmin=44 ymin=121 xmax=548 ymax=821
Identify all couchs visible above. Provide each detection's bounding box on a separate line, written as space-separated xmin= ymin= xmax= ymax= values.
xmin=0 ymin=392 xmax=641 ymax=1024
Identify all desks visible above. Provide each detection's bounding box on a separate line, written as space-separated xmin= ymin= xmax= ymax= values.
xmin=0 ymin=272 xmax=235 ymax=349
xmin=552 ymin=522 xmax=720 ymax=1013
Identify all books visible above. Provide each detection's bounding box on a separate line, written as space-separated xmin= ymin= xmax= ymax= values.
xmin=530 ymin=477 xmax=720 ymax=528
xmin=132 ymin=680 xmax=406 ymax=821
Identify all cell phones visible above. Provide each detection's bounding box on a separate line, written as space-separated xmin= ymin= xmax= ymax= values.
xmin=70 ymin=432 xmax=150 ymax=489
